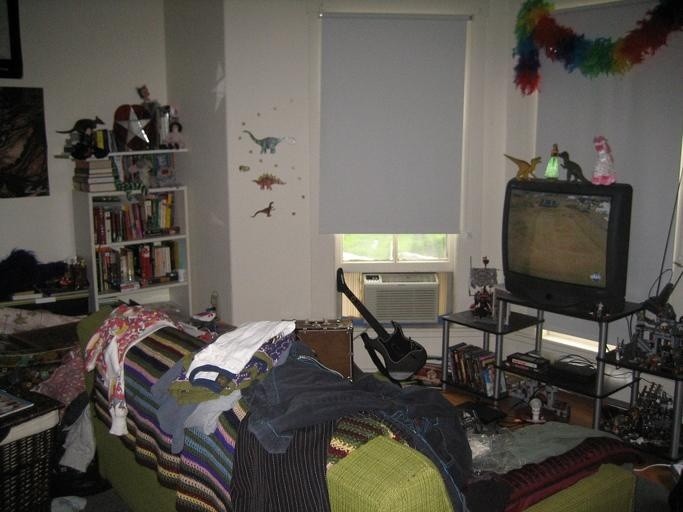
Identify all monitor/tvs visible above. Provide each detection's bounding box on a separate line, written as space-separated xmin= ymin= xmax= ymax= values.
xmin=501 ymin=177 xmax=633 ymax=317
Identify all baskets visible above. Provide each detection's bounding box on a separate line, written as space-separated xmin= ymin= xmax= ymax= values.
xmin=0 ymin=384 xmax=66 ymax=512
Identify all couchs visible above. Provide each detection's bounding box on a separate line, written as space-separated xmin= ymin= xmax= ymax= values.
xmin=76 ymin=306 xmax=638 ymax=511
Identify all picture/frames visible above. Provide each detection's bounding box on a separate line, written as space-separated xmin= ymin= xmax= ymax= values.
xmin=0 ymin=0 xmax=24 ymax=80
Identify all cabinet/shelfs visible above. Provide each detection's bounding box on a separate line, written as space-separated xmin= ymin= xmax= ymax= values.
xmin=71 ymin=146 xmax=193 ymax=317
xmin=438 ymin=279 xmax=682 ymax=461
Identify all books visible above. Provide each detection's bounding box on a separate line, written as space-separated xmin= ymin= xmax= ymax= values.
xmin=12 ymin=293 xmax=42 ymax=300
xmin=0 ymin=295 xmax=56 ymax=307
xmin=70 ymin=154 xmax=186 ymax=294
xmin=14 ymin=290 xmax=34 ymax=295
xmin=0 ymin=389 xmax=33 ymax=416
xmin=445 ymin=341 xmax=551 ymax=400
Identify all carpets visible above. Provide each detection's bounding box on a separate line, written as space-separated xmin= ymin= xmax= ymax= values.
xmin=634 ymin=472 xmax=674 ymax=512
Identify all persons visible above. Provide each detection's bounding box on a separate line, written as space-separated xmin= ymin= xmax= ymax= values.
xmin=592 ymin=135 xmax=617 ymax=185
xmin=542 ymin=144 xmax=559 ymax=179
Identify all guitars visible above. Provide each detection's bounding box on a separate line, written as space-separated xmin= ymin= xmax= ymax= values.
xmin=336 ymin=268 xmax=428 ymax=382
